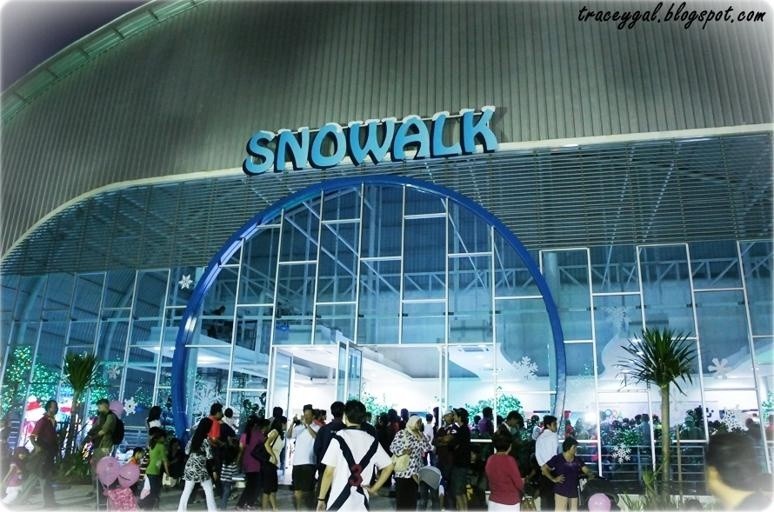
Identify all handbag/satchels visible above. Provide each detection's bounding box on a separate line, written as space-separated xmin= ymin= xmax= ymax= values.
xmin=250 ymin=440 xmax=271 ymax=465
xmin=105 ymin=411 xmax=124 ymax=445
xmin=521 ymin=489 xmax=536 ymax=511
xmin=392 ymin=454 xmax=409 ymax=470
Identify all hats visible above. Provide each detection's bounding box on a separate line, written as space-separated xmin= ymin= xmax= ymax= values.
xmin=453 ymin=408 xmax=468 ymax=419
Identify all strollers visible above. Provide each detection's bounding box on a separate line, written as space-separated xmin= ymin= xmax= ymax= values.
xmin=97 ymin=467 xmax=141 ymax=512
xmin=575 ymin=473 xmax=621 ymax=512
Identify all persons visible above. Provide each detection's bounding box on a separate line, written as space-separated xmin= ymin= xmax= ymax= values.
xmin=1 ymin=398 xmax=774 ymax=511
